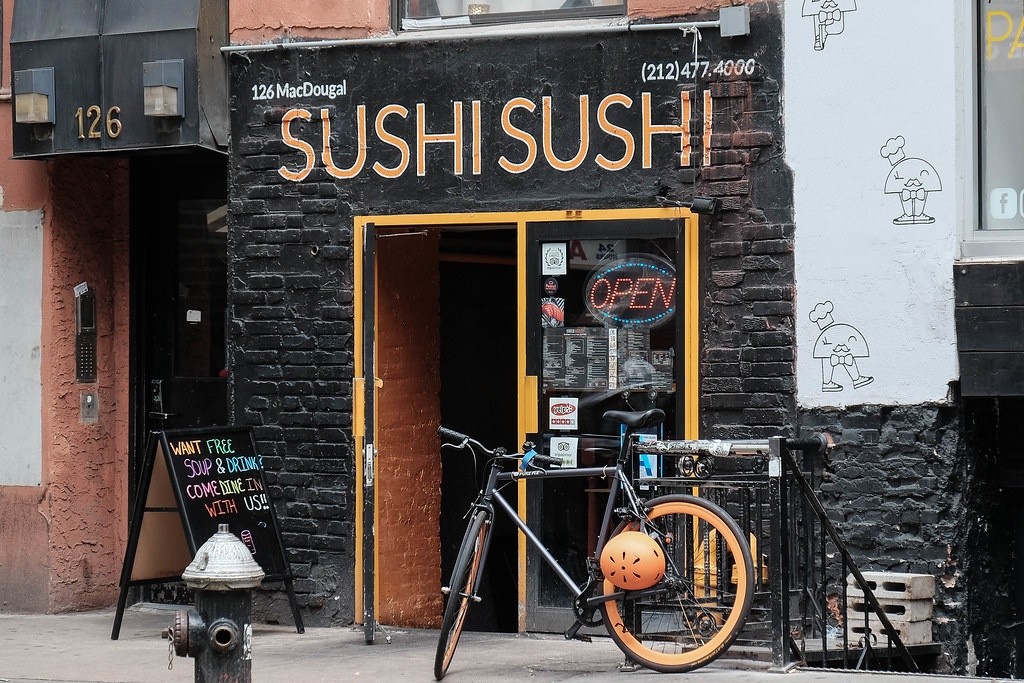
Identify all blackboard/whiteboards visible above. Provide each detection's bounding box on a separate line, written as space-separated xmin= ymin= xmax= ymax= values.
xmin=156 ymin=421 xmax=291 ymax=585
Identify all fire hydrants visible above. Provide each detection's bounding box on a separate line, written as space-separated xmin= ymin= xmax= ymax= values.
xmin=160 ymin=524 xmax=263 ymax=683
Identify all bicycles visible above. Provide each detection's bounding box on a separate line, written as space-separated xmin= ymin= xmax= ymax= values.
xmin=433 ymin=409 xmax=756 ymax=682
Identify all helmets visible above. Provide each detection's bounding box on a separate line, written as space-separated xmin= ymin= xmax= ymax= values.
xmin=600 ymin=531 xmax=666 ymax=591
